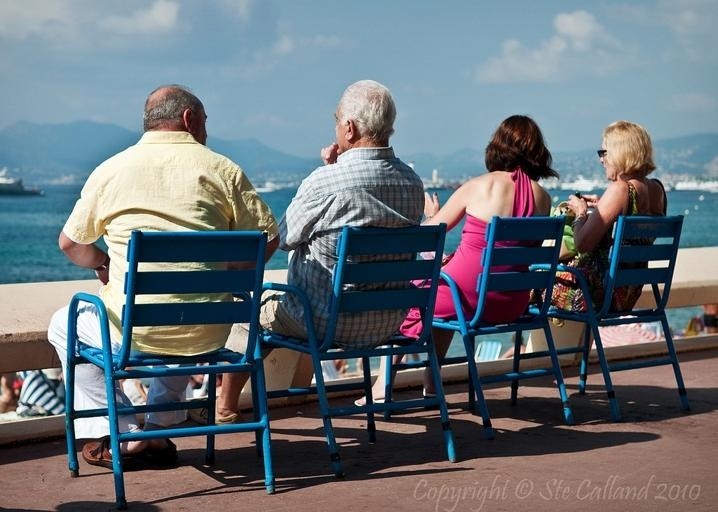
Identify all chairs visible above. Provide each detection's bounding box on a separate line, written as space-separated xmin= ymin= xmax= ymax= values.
xmin=60 ymin=229 xmax=274 ymax=508
xmin=473 ymin=341 xmax=501 ymax=361
xmin=387 ymin=215 xmax=584 ymax=437
xmin=258 ymin=222 xmax=461 ymax=478
xmin=503 ymin=215 xmax=697 ymax=425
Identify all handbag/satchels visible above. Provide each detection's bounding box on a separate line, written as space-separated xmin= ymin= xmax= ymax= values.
xmin=543 ymin=202 xmax=578 ymax=261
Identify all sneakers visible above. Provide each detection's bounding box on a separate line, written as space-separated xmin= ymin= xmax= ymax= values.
xmin=188 ymin=397 xmax=242 ymax=424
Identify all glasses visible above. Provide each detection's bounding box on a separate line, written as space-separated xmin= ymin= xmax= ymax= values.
xmin=597 ymin=150 xmax=607 ymax=157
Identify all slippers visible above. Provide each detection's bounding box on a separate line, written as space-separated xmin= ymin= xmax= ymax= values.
xmin=354 ymin=395 xmax=395 ymax=407
xmin=423 ymin=388 xmax=437 ymax=398
xmin=83 ymin=437 xmax=179 ymax=470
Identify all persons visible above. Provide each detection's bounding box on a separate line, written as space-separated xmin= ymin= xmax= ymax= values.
xmin=47 ymin=84 xmax=279 ymax=473
xmin=354 ymin=115 xmax=560 ymax=407
xmin=335 ymin=353 xmax=420 ymax=378
xmin=0 ymin=358 xmax=223 ymax=417
xmin=187 ymin=79 xmax=424 ymax=426
xmin=656 ymin=303 xmax=718 ymax=339
xmin=500 ymin=333 xmax=526 ymax=359
xmin=530 ymin=120 xmax=667 ymax=319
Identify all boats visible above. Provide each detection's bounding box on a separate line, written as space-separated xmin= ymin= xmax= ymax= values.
xmin=537 ymin=174 xmax=609 ymax=191
xmin=253 ymin=180 xmax=294 ymax=195
xmin=408 ymin=160 xmax=465 ymax=189
xmin=663 ymin=175 xmax=718 ymax=192
xmin=0 ymin=167 xmax=44 ymax=196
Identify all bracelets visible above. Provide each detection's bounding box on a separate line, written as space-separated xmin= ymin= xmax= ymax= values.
xmin=570 ymin=213 xmax=586 ymax=232
xmin=593 ymin=199 xmax=598 ymax=210
xmin=426 ymin=216 xmax=433 ymax=219
xmin=93 ymin=254 xmax=111 ymax=272
